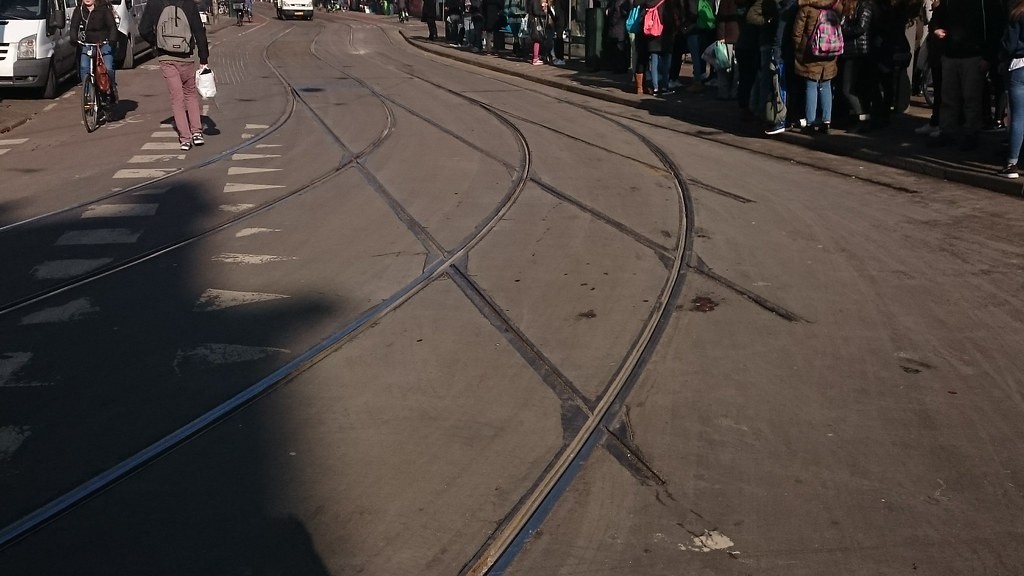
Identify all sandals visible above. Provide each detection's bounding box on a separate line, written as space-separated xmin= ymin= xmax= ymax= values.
xmin=634 ymin=72 xmax=645 ymax=95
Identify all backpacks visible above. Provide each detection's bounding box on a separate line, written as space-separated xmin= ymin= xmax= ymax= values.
xmin=155 ymin=0 xmax=192 ymax=55
xmin=809 ymin=10 xmax=844 ymax=58
xmin=644 ymin=0 xmax=663 ymax=36
xmin=626 ymin=4 xmax=643 ymax=33
xmin=697 ymin=0 xmax=715 ymax=29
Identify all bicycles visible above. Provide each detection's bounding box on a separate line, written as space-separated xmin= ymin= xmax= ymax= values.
xmin=76 ymin=39 xmax=119 ymax=133
xmin=198 ymin=0 xmax=253 ymax=28
xmin=399 ymin=10 xmax=406 ymax=24
xmin=316 ymin=0 xmax=350 ymax=15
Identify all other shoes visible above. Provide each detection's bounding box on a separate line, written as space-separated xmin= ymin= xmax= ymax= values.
xmin=533 ymin=59 xmax=543 ymax=66
xmin=914 ymin=122 xmax=939 ymax=137
xmin=765 ymin=128 xmax=785 ymax=134
xmin=912 ymin=89 xmax=923 ymax=96
xmin=801 ymin=126 xmax=815 ymax=133
xmin=653 ymin=90 xmax=659 ymax=96
xmin=701 ymin=75 xmax=718 ymax=85
xmin=686 ymin=84 xmax=705 ymax=92
xmin=785 ymin=124 xmax=793 ymax=130
xmin=553 ymin=59 xmax=564 ymax=64
xmin=983 ymin=121 xmax=1006 ymax=133
xmin=818 ymin=124 xmax=830 ymax=134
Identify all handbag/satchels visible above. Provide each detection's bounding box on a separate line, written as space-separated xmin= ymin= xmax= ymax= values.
xmin=661 ymin=89 xmax=675 ymax=95
xmin=760 ymin=68 xmax=787 ymax=124
xmin=94 ymin=45 xmax=110 ymax=92
xmin=531 ymin=16 xmax=546 ymax=43
xmin=195 ymin=64 xmax=216 ymax=97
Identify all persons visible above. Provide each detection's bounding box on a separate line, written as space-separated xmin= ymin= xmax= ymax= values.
xmin=138 ymin=0 xmax=209 ymax=150
xmin=395 ymin=0 xmax=1024 ymax=177
xmin=70 ymin=0 xmax=119 ymax=104
xmin=236 ymin=0 xmax=252 ymax=24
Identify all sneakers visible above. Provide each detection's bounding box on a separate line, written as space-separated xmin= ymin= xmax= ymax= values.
xmin=192 ymin=131 xmax=205 ymax=144
xmin=180 ymin=141 xmax=190 ymax=150
xmin=997 ymin=162 xmax=1020 ymax=179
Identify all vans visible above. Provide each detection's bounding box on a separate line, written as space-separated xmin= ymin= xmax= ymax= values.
xmin=274 ymin=0 xmax=314 ymax=21
xmin=0 ymin=0 xmax=147 ymax=99
